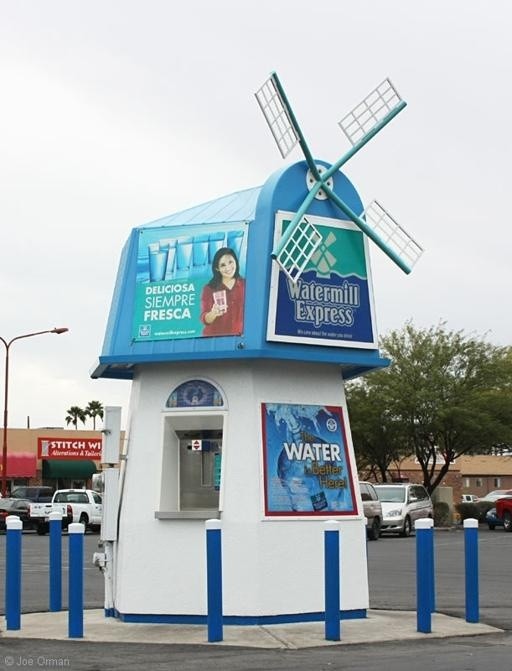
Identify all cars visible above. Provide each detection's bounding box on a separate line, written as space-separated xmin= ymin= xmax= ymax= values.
xmin=472 ymin=489 xmax=512 ymax=531
xmin=0 ymin=485 xmax=57 ymax=536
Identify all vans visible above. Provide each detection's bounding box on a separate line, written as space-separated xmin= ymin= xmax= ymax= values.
xmin=359 ymin=480 xmax=436 ymax=540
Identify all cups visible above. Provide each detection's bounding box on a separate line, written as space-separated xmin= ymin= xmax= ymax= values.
xmin=177 ymin=235 xmax=194 ymax=270
xmin=227 ymin=230 xmax=244 ymax=261
xmin=148 ymin=242 xmax=170 ymax=283
xmin=208 ymin=231 xmax=225 ymax=265
xmin=159 ymin=239 xmax=177 ymax=276
xmin=192 ymin=232 xmax=210 ymax=267
xmin=213 ymin=289 xmax=227 ymax=315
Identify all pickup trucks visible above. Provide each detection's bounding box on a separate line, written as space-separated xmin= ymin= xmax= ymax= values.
xmin=28 ymin=487 xmax=102 ymax=536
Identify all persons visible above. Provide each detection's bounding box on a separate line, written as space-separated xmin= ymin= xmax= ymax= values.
xmin=199 ymin=247 xmax=245 ymax=337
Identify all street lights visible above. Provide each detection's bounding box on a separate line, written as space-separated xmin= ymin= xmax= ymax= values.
xmin=0 ymin=326 xmax=70 ymax=494
